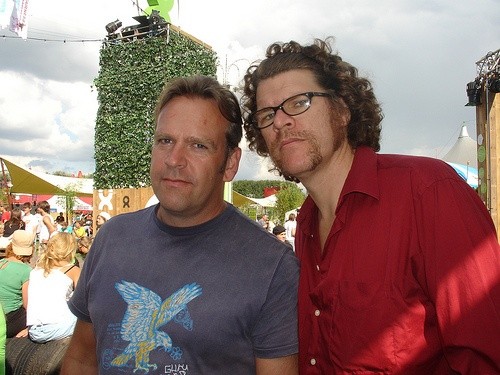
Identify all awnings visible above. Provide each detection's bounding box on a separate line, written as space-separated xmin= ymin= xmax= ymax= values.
xmin=233 ymin=181 xmax=309 ymax=214
xmin=0 ymin=156 xmax=95 ymax=212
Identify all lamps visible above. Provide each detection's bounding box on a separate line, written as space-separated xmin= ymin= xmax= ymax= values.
xmin=464 ymin=81 xmax=484 ymax=106
xmin=105 ymin=19 xmax=122 ymax=35
xmin=487 ymin=77 xmax=500 ymax=92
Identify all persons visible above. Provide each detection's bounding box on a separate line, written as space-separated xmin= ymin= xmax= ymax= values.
xmin=0 ymin=230 xmax=36 ymax=338
xmin=0 ymin=202 xmax=111 ymax=270
xmin=240 ymin=37 xmax=500 ymax=375
xmin=254 ymin=213 xmax=298 ymax=255
xmin=60 ymin=76 xmax=300 ymax=375
xmin=26 ymin=232 xmax=82 ymax=345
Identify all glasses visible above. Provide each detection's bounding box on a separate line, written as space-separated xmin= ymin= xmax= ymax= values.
xmin=250 ymin=92 xmax=337 ymax=130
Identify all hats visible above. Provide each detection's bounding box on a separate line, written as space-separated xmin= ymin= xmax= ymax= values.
xmin=12 ymin=230 xmax=34 ymax=256
xmin=100 ymin=212 xmax=112 ymax=221
